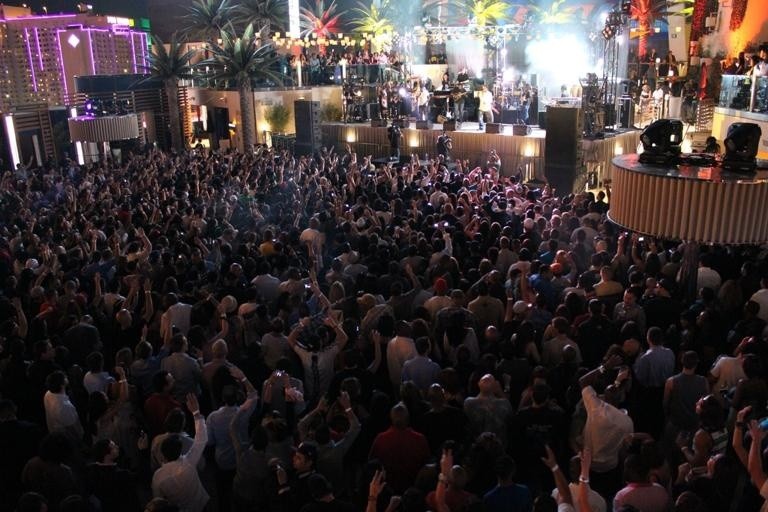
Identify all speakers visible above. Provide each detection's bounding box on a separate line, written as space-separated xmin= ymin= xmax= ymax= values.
xmin=364 ymin=64 xmax=389 ymax=84
xmin=324 ymin=65 xmax=344 ymax=86
xmin=367 ymin=103 xmax=379 ymax=119
xmin=206 ymin=107 xmax=230 ymax=149
xmin=618 ymin=98 xmax=635 ymax=129
xmin=544 ymin=107 xmax=583 ymax=196
xmin=293 ymin=100 xmax=323 ymax=152
xmin=346 ymin=63 xmax=365 ymax=86
xmin=48 ymin=105 xmax=76 ymax=167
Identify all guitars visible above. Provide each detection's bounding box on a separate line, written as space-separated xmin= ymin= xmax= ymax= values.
xmin=450 ymin=90 xmax=473 ymax=102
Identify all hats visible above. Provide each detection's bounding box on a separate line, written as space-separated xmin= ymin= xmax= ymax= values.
xmin=220 ymin=294 xmax=238 ymax=312
xmin=434 ymin=279 xmax=448 ymax=290
xmin=289 ymin=442 xmax=322 ymax=460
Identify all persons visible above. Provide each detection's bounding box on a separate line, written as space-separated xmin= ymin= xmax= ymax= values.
xmin=416 ymin=86 xmax=430 ymax=121
xmin=627 ymin=47 xmax=679 ymax=77
xmin=387 ymin=127 xmax=404 ymax=156
xmin=653 ymin=83 xmax=663 ymax=119
xmin=561 ymin=86 xmax=569 ymax=97
xmin=451 ymin=83 xmax=467 ymax=125
xmin=441 ymin=73 xmax=451 ymax=89
xmin=427 ymin=48 xmax=449 ymax=64
xmin=704 ymin=136 xmax=720 ymax=156
xmin=436 ymin=134 xmax=452 ymax=155
xmin=286 ymin=50 xmax=396 ymax=84
xmin=456 ymin=68 xmax=469 ymax=90
xmin=637 ymin=84 xmax=652 ymax=114
xmin=728 ymin=41 xmax=768 ymax=78
xmin=473 ymin=84 xmax=494 ymax=131
xmin=516 ymin=74 xmax=527 ymax=88
xmin=517 ymin=84 xmax=533 ymax=124
